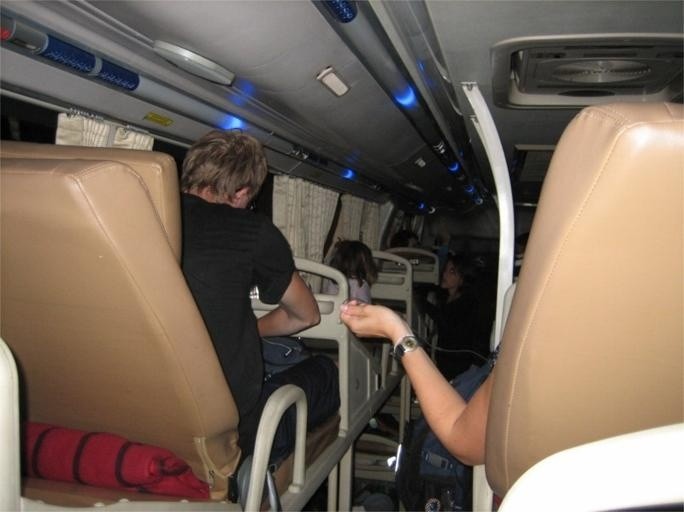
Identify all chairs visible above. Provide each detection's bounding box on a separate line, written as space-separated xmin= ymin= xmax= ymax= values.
xmin=480 ymin=102 xmax=684 ymax=511
xmin=0 ymin=139 xmax=440 ymax=512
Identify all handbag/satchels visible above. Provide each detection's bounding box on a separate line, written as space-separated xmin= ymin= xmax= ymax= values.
xmin=260 ymin=335 xmax=311 ymax=380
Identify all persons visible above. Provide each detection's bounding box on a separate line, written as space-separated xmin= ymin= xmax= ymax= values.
xmin=182 ymin=130 xmax=340 ymax=458
xmin=327 ymin=229 xmax=495 ymax=465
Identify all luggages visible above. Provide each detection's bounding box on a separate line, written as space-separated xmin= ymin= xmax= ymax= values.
xmin=393 ymin=349 xmax=496 ymax=512
